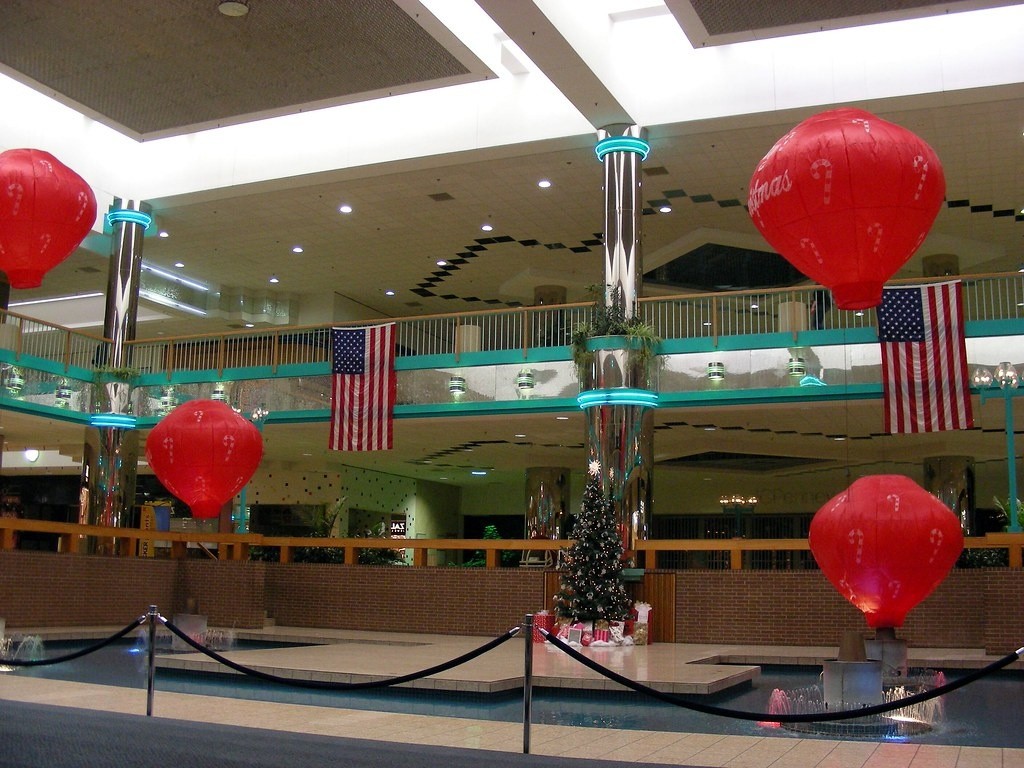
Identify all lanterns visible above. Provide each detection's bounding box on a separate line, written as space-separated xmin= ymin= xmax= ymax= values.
xmin=749 ymin=107 xmax=946 ymax=312
xmin=810 ymin=474 xmax=963 ymax=630
xmin=145 ymin=399 xmax=262 ymax=519
xmin=0 ymin=147 xmax=99 ymax=289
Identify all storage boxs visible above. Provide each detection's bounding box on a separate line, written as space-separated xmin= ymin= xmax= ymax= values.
xmin=568 ymin=608 xmax=654 ymax=649
xmin=533 ymin=610 xmax=555 ymax=643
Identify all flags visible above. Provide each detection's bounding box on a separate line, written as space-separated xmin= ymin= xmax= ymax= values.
xmin=329 ymin=320 xmax=400 ymax=451
xmin=876 ymin=278 xmax=975 ymax=433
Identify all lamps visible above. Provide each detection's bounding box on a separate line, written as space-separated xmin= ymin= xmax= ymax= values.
xmin=786 ymin=358 xmax=806 ymax=377
xmin=708 ymin=362 xmax=725 ymax=381
xmin=160 ymin=396 xmax=176 ymax=406
xmin=59 ymin=386 xmax=72 ymax=399
xmin=516 ymin=369 xmax=535 ymax=389
xmin=449 ymin=377 xmax=467 ymax=394
xmin=210 ymin=389 xmax=225 ymax=403
xmin=10 ymin=375 xmax=25 ymax=390
